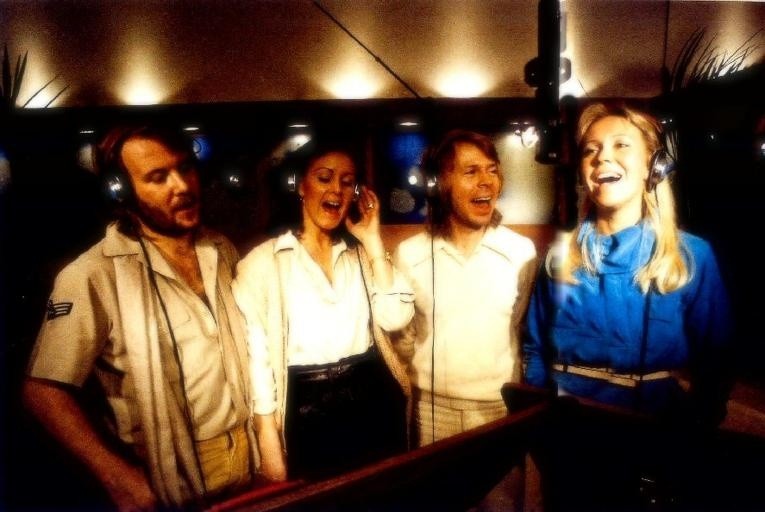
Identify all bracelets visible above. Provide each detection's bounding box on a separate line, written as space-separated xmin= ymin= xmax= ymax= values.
xmin=366 ymin=252 xmax=393 ymax=266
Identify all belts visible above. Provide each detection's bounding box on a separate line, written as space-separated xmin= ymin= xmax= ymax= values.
xmin=293 ymin=360 xmax=355 ymax=382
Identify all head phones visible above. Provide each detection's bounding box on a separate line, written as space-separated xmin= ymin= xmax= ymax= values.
xmin=423 ymin=142 xmax=441 ymax=198
xmin=102 ymin=127 xmax=133 ymax=209
xmin=648 ymin=109 xmax=677 ymax=185
xmin=286 ymin=139 xmax=362 ymax=203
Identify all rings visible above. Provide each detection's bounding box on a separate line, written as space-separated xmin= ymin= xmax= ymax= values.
xmin=366 ymin=204 xmax=374 ymax=209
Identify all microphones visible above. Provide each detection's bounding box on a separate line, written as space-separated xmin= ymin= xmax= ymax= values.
xmin=534 ymin=115 xmax=562 ymax=164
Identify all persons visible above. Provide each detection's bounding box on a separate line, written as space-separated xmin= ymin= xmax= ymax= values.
xmin=390 ymin=125 xmax=540 ymax=450
xmin=226 ymin=132 xmax=417 ymax=482
xmin=21 ymin=106 xmax=259 ymax=510
xmin=524 ymin=99 xmax=740 ymax=510
